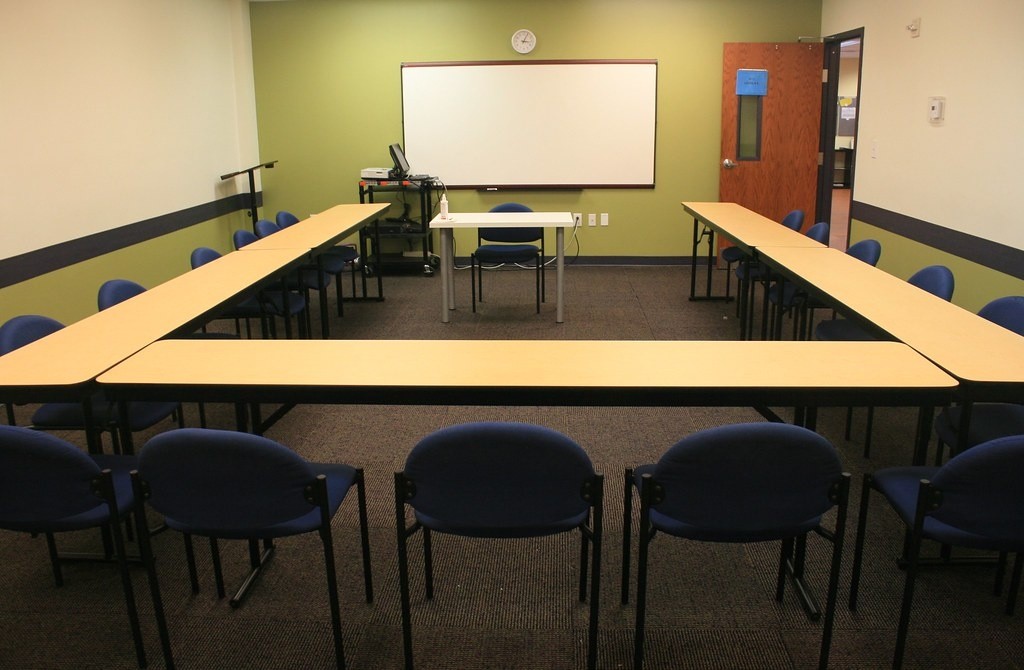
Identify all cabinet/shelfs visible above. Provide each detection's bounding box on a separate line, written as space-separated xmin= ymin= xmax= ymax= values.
xmin=358 ymin=176 xmax=440 ymax=275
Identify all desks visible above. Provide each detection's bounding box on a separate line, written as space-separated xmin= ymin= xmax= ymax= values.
xmin=238 ymin=203 xmax=391 ymax=338
xmin=679 ymin=199 xmax=829 ymax=344
xmin=93 ymin=337 xmax=960 ymax=670
xmin=429 ymin=212 xmax=575 ymax=323
xmin=1 ymin=249 xmax=312 ymax=534
xmin=751 ymin=246 xmax=1024 ymax=465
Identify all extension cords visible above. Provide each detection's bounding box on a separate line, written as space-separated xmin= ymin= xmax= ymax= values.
xmin=403 ymin=251 xmax=431 ymax=257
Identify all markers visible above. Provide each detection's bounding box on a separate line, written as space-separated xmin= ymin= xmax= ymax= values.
xmin=486 ymin=188 xmax=497 ymax=190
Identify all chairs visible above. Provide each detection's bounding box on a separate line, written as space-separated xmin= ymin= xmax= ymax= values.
xmin=0 ymin=211 xmax=364 ymax=562
xmin=469 ymin=202 xmax=546 ymax=315
xmin=722 ymin=210 xmax=1024 ymax=465
xmin=0 ymin=419 xmax=1024 ymax=670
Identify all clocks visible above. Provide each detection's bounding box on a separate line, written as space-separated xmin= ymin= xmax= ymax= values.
xmin=511 ymin=29 xmax=537 ymax=55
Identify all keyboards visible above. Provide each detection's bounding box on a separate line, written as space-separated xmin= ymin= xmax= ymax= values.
xmin=410 ymin=175 xmax=428 ymax=182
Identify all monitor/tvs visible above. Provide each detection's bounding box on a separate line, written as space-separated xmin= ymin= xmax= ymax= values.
xmin=389 ymin=144 xmax=410 ymax=174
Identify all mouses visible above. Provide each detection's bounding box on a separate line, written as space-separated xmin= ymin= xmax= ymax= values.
xmin=409 ymin=175 xmax=413 ymax=177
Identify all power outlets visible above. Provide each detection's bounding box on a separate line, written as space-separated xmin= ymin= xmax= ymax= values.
xmin=572 ymin=213 xmax=582 ymax=227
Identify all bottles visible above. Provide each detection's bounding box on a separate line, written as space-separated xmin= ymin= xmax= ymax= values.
xmin=440 ymin=194 xmax=449 ymax=219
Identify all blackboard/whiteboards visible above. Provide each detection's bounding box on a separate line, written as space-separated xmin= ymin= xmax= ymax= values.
xmin=401 ymin=58 xmax=657 ymax=191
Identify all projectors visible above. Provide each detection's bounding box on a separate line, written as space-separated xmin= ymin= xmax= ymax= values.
xmin=360 ymin=168 xmax=393 ymax=179
